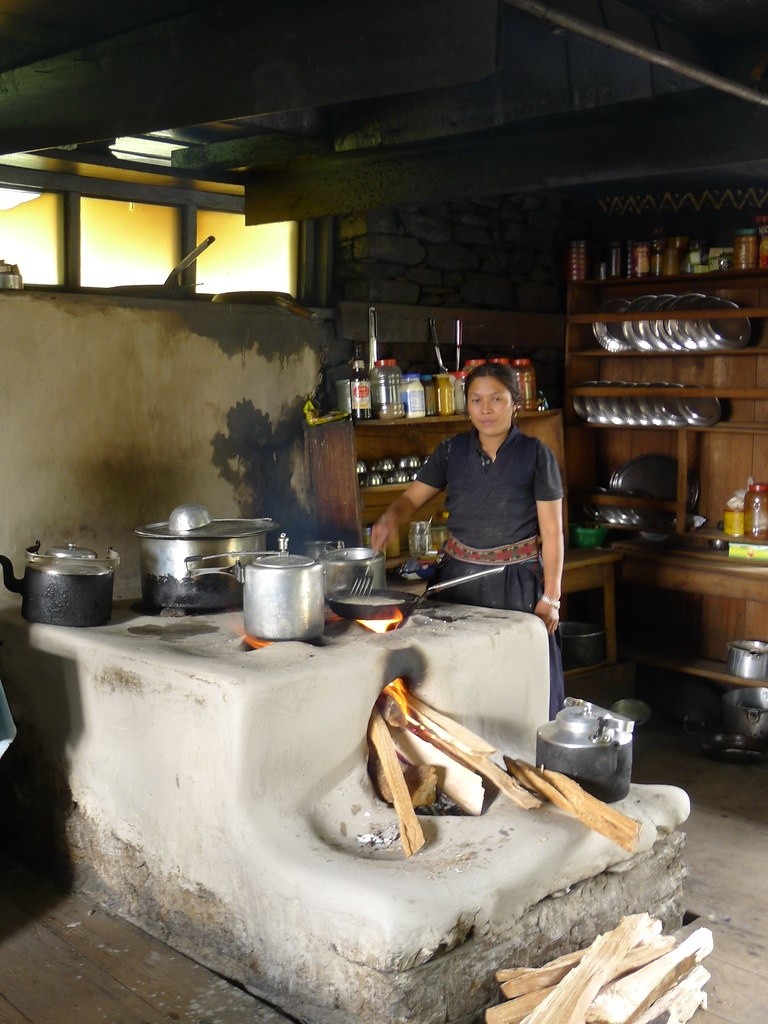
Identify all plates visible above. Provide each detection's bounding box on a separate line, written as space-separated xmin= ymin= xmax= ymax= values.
xmin=582 ymin=486 xmax=618 ymax=524
xmin=592 ymin=293 xmax=751 ymax=352
xmin=573 ymin=381 xmax=721 ymax=426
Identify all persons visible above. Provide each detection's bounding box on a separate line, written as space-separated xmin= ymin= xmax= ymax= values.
xmin=372 ymin=361 xmax=566 ymax=721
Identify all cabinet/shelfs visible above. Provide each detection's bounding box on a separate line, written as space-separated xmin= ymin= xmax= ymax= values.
xmin=303 ymin=408 xmax=568 ymax=624
xmin=560 ymin=234 xmax=768 ymax=687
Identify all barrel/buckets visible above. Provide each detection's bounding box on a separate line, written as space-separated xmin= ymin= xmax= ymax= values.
xmin=559 ymin=621 xmax=607 ymax=670
xmin=335 ymin=379 xmax=352 ymax=414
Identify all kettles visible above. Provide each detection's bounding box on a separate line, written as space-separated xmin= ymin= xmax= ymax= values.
xmin=0 ymin=260 xmax=24 ymax=289
xmin=537 ymin=697 xmax=635 ymax=803
xmin=0 ymin=539 xmax=120 ymax=627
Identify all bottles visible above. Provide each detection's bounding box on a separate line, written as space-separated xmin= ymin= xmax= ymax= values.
xmin=431 ymin=526 xmax=448 ymax=551
xmin=744 ymin=484 xmax=768 ymax=540
xmin=350 ymin=345 xmax=537 ymax=421
xmin=409 ymin=521 xmax=432 ymax=557
xmin=600 ymin=215 xmax=768 ymax=281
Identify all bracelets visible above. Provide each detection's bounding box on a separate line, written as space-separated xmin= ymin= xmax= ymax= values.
xmin=541 ymin=594 xmax=561 ymax=610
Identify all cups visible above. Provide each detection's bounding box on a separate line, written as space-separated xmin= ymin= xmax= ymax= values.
xmin=565 ymin=240 xmax=588 ymax=280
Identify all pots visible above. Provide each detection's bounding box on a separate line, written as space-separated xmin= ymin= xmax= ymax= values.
xmin=710 ymin=733 xmax=768 ymax=764
xmin=318 ymin=547 xmax=386 ymax=594
xmin=212 ymin=291 xmax=319 ymax=321
xmin=110 ymin=235 xmax=216 ymax=298
xmin=184 ymin=533 xmax=326 ymax=641
xmin=325 ymin=564 xmax=507 ymax=620
xmin=302 ymin=540 xmax=345 ymax=562
xmin=722 ymin=687 xmax=768 ymax=743
xmin=133 ymin=517 xmax=280 ymax=608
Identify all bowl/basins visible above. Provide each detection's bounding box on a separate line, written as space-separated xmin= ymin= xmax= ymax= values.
xmin=708 ymin=540 xmax=729 ymax=550
xmin=728 ymin=640 xmax=768 ymax=679
xmin=608 ymin=698 xmax=652 ymax=725
xmin=357 ymin=456 xmax=430 ymax=486
xmin=569 ymin=524 xmax=608 ymax=549
